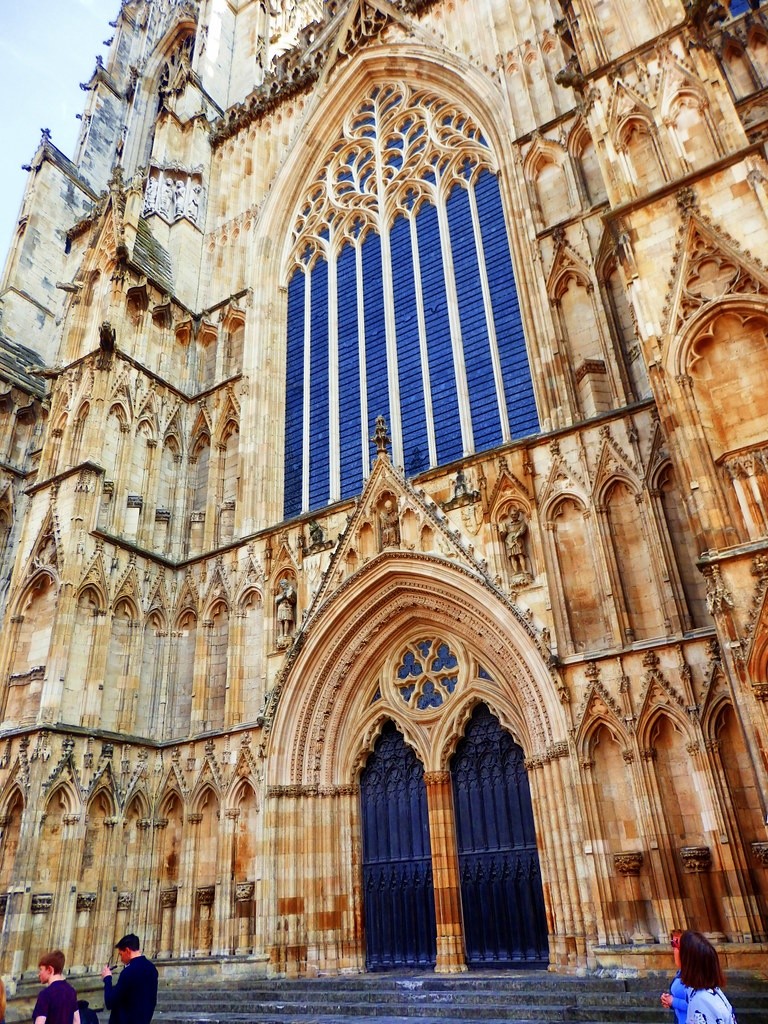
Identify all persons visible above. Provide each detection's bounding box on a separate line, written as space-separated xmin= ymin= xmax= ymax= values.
xmin=98 ymin=321 xmax=116 ymax=369
xmin=661 ymin=930 xmax=738 ymax=1024
xmin=0 ymin=979 xmax=6 ymax=1024
xmin=501 ymin=510 xmax=530 ymax=574
xmin=102 ymin=934 xmax=159 ymax=1024
xmin=274 ymin=579 xmax=296 ymax=637
xmin=32 ymin=951 xmax=82 ymax=1024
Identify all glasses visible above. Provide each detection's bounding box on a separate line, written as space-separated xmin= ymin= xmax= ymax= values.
xmin=671 ymin=939 xmax=680 ymax=950
